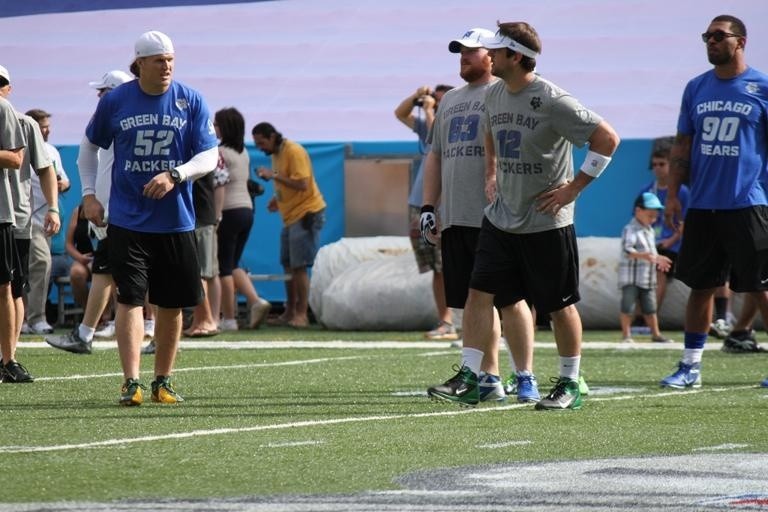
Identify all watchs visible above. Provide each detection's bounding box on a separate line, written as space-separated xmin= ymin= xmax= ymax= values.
xmin=272 ymin=170 xmax=279 ymax=179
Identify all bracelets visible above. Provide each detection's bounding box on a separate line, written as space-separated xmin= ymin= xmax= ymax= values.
xmin=580 ymin=150 xmax=612 ymax=180
xmin=272 ymin=194 xmax=277 ymax=198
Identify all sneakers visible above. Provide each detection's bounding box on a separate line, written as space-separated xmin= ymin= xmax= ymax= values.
xmin=0 ymin=357 xmax=34 ymax=382
xmin=428 ymin=365 xmax=589 ymax=410
xmin=21 ymin=320 xmax=54 ymax=334
xmin=183 ymin=296 xmax=310 ymax=337
xmin=660 ymin=361 xmax=703 ymax=389
xmin=152 ymin=376 xmax=183 ymax=402
xmin=141 ymin=336 xmax=181 ymax=352
xmin=143 ymin=319 xmax=155 ymax=337
xmin=708 ymin=314 xmax=768 ymax=353
xmin=119 ymin=377 xmax=148 ymax=405
xmin=46 ymin=324 xmax=92 ymax=354
xmin=426 ymin=320 xmax=459 ymax=340
xmin=94 ymin=320 xmax=116 ymax=338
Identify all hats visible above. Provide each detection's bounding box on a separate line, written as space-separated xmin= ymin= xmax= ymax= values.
xmin=479 ymin=26 xmax=537 ymax=59
xmin=91 ymin=71 xmax=133 ymax=89
xmin=635 ymin=192 xmax=665 ymax=208
xmin=448 ymin=28 xmax=495 ymax=53
xmin=130 ymin=31 xmax=174 ymax=76
xmin=0 ymin=65 xmax=10 ymax=82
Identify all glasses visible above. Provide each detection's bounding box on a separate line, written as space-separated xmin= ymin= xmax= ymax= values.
xmin=701 ymin=31 xmax=744 ymax=42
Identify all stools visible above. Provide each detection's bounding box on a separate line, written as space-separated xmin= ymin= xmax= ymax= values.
xmin=53 ymin=276 xmax=83 ymax=328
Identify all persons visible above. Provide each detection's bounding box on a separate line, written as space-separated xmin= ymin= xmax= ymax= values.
xmin=393 ymin=84 xmax=460 ymax=341
xmin=425 ymin=22 xmax=621 ymax=411
xmin=418 ymin=27 xmax=541 ymax=404
xmin=502 ymin=65 xmax=590 ymax=395
xmin=252 ymin=122 xmax=326 ymax=330
